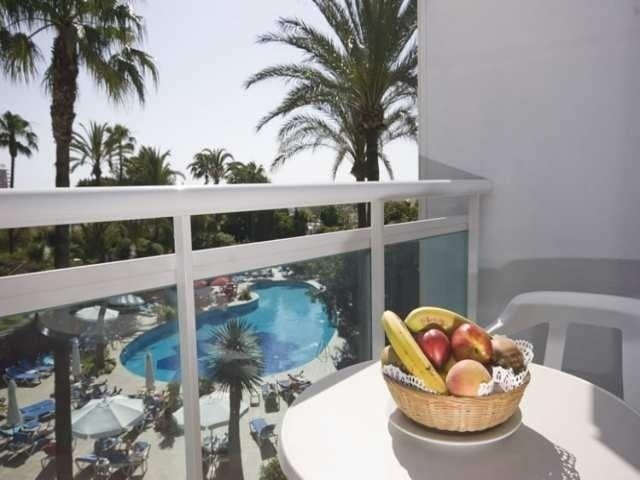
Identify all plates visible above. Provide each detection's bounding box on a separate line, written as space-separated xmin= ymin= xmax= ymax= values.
xmin=386 ymin=408 xmax=524 ymax=447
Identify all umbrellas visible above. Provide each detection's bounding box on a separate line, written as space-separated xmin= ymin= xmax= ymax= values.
xmin=72 ymin=304 xmax=121 ymax=323
xmin=209 ymin=277 xmax=234 ymax=287
xmin=66 ymin=392 xmax=150 ymax=442
xmin=108 ymin=294 xmax=147 ymax=307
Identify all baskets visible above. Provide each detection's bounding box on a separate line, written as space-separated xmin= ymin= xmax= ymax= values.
xmin=382 ymin=366 xmax=531 ymax=432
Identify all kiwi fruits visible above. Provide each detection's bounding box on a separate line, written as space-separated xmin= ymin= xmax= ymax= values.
xmin=489 ymin=335 xmax=524 ymax=372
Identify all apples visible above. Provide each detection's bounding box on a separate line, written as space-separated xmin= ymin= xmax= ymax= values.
xmin=446 ymin=359 xmax=492 ymax=397
xmin=414 ymin=328 xmax=452 ymax=371
xmin=451 ymin=323 xmax=493 ymax=367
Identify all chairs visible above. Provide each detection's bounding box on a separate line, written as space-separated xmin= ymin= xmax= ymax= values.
xmin=73 ymin=436 xmax=152 ymax=480
xmin=481 ymin=288 xmax=640 ymax=414
xmin=201 ymin=433 xmax=229 ymax=472
xmin=11 ymin=428 xmax=54 ymax=458
xmin=4 ymin=352 xmax=55 ymax=385
xmin=248 ymin=417 xmax=277 ymax=446
xmin=259 ymin=373 xmax=310 ymax=403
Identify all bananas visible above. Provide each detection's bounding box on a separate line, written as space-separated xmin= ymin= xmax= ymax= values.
xmin=380 ymin=309 xmax=447 ymax=394
xmin=403 ymin=305 xmax=469 ymax=333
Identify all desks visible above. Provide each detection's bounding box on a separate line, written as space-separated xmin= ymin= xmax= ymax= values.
xmin=275 ymin=357 xmax=640 ymax=480
